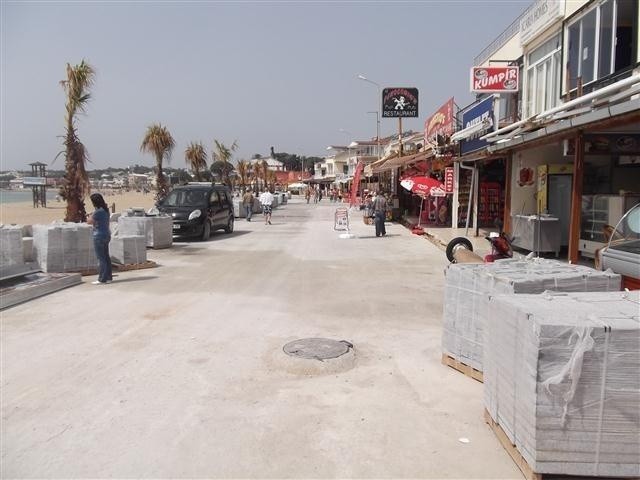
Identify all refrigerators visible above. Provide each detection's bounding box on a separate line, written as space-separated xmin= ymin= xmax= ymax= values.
xmin=480 ymin=182 xmax=500 ymax=227
xmin=536 ymin=163 xmax=574 ymax=246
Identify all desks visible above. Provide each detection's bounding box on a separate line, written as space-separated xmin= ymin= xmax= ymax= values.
xmin=512 ymin=215 xmax=561 ymax=257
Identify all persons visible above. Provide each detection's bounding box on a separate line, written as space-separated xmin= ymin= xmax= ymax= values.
xmin=373 ymin=191 xmax=386 ymax=236
xmin=85 ymin=193 xmax=114 ymax=286
xmin=240 ymin=189 xmax=255 ymax=222
xmin=360 ymin=188 xmax=392 ymax=222
xmin=259 ymin=186 xmax=276 ymax=226
xmin=300 ymin=185 xmax=349 ymax=207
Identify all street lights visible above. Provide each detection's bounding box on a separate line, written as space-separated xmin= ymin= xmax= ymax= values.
xmin=358 ymin=74 xmax=381 ymax=195
xmin=337 ymin=127 xmax=352 ymax=200
xmin=296 ymin=155 xmax=305 ymax=185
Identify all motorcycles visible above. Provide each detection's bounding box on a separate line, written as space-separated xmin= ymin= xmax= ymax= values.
xmin=484 ymin=233 xmax=516 ymax=264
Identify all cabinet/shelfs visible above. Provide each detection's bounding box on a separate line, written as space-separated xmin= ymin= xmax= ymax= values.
xmin=578 ymin=191 xmax=640 ymax=292
xmin=458 ymin=183 xmax=471 ymax=226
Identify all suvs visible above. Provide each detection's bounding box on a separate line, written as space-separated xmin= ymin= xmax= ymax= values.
xmin=152 ymin=180 xmax=236 ymax=243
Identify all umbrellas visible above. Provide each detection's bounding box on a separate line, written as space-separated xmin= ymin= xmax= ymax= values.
xmin=399 ymin=175 xmax=448 ymax=228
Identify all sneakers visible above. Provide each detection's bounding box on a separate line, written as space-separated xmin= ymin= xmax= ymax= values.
xmin=92 ymin=280 xmax=111 ymax=285
